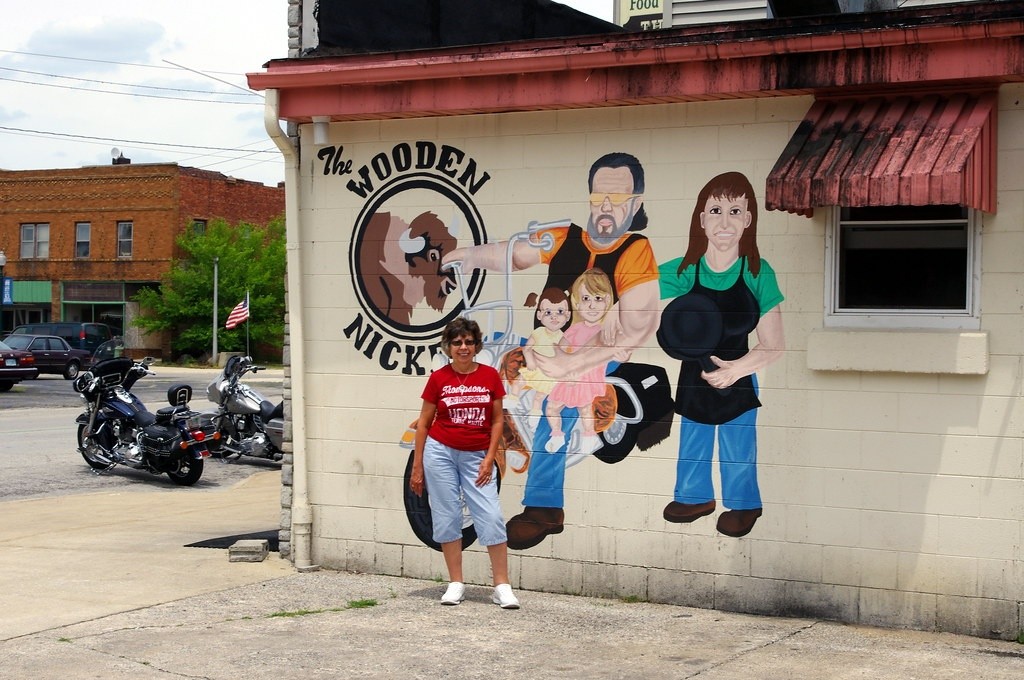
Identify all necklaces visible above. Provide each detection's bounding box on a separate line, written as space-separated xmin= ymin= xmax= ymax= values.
xmin=453 ymin=366 xmax=467 ymax=390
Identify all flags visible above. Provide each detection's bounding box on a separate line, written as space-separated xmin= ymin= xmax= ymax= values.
xmin=226 ymin=292 xmax=248 ymax=329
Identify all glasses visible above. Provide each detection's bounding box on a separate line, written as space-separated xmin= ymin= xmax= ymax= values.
xmin=450 ymin=338 xmax=476 ymax=346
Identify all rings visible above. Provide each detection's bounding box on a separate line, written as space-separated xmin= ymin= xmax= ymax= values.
xmin=489 ymin=476 xmax=491 ymax=479
xmin=412 ymin=485 xmax=414 ymax=487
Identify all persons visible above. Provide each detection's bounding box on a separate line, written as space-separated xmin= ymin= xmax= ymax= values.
xmin=410 ymin=317 xmax=519 ymax=608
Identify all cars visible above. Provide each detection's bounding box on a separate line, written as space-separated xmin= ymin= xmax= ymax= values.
xmin=0 ymin=334 xmax=91 ymax=381
xmin=0 ymin=341 xmax=40 ymax=393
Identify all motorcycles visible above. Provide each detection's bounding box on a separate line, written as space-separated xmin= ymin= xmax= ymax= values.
xmin=193 ymin=354 xmax=284 ymax=463
xmin=72 ymin=338 xmax=221 ymax=485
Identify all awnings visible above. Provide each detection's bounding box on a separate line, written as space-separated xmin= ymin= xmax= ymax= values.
xmin=764 ymin=93 xmax=997 ymax=218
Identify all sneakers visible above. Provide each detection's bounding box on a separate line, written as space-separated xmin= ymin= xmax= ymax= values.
xmin=493 ymin=583 xmax=520 ymax=607
xmin=440 ymin=582 xmax=466 ymax=605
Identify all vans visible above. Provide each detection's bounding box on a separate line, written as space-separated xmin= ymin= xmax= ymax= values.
xmin=3 ymin=322 xmax=113 ymax=371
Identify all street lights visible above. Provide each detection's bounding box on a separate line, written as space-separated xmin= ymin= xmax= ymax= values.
xmin=0 ymin=251 xmax=7 ymax=337
